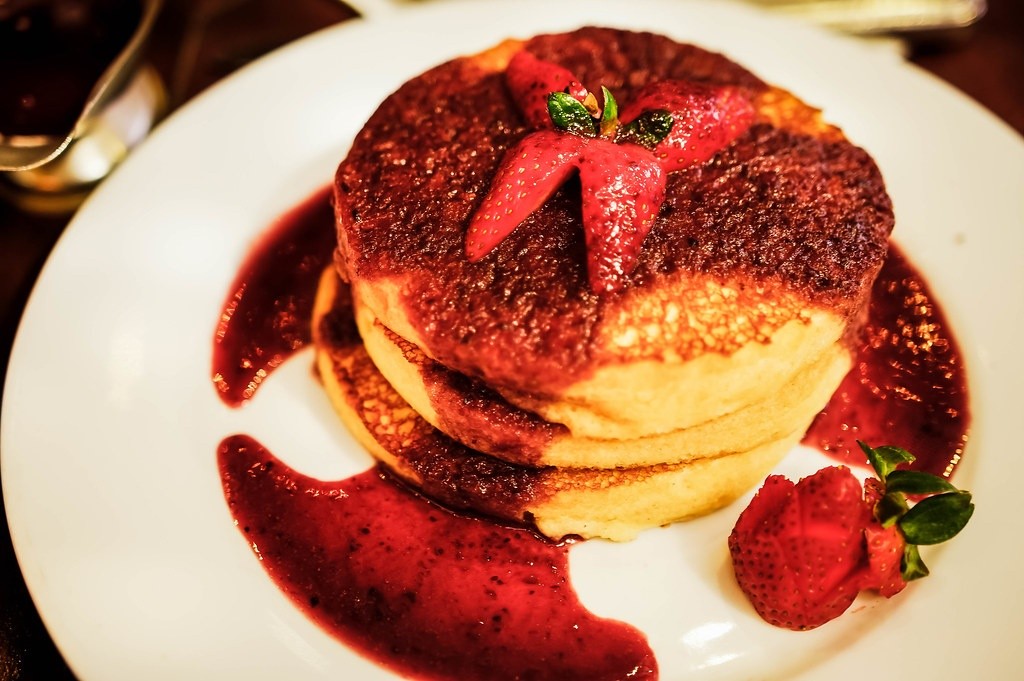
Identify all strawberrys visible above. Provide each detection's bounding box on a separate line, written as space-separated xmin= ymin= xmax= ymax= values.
xmin=728 ymin=440 xmax=978 ymax=629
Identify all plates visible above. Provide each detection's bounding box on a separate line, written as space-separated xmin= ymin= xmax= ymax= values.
xmin=0 ymin=0 xmax=1024 ymax=681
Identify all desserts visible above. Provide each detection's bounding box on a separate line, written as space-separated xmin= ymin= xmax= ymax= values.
xmin=309 ymin=26 xmax=896 ymax=540
